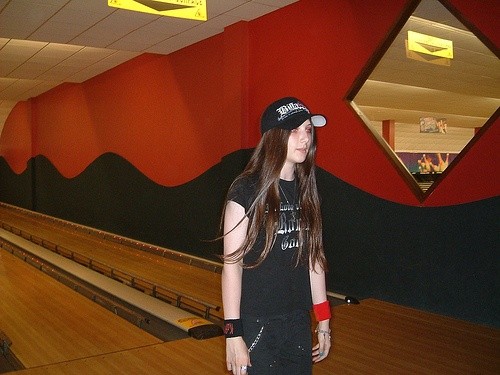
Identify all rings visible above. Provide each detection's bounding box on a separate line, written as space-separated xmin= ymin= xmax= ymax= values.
xmin=240 ymin=366 xmax=247 ymax=370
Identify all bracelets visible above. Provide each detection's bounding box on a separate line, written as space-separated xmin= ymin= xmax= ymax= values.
xmin=312 ymin=300 xmax=332 ymax=322
xmin=315 ymin=326 xmax=331 ymax=340
xmin=223 ymin=319 xmax=243 ymax=338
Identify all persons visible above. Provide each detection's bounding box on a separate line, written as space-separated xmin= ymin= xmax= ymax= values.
xmin=200 ymin=96 xmax=333 ymax=375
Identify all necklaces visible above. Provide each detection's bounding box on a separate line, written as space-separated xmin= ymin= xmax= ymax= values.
xmin=278 ymin=177 xmax=296 ymax=222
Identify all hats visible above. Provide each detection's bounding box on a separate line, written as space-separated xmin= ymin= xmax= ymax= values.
xmin=261 ymin=97 xmax=327 ymax=136
